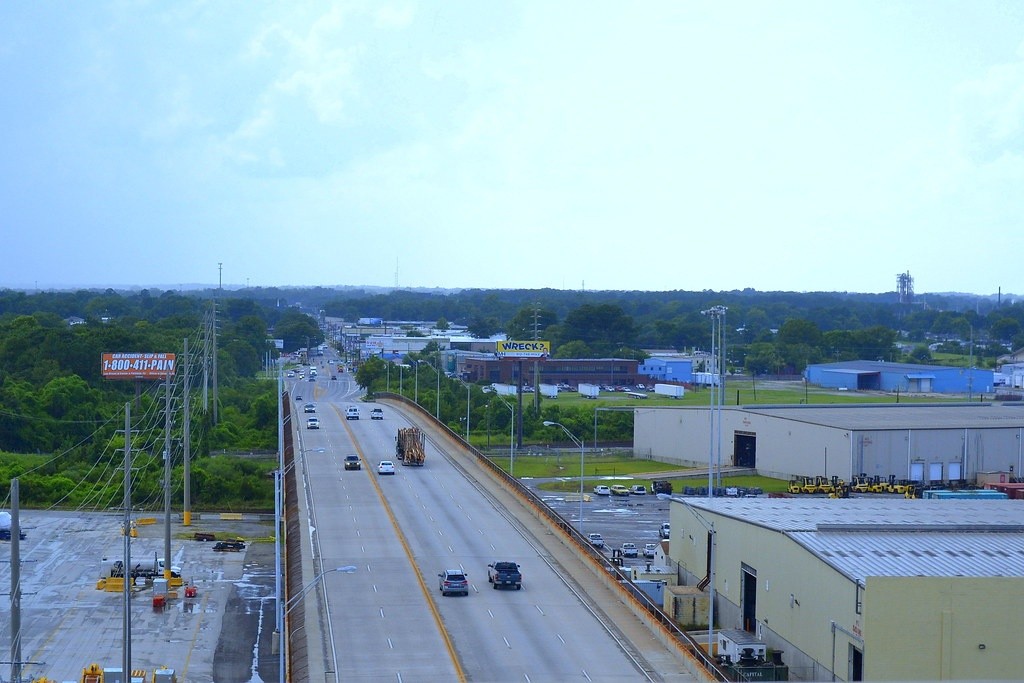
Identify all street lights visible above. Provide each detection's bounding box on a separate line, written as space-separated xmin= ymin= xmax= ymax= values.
xmin=361 ymin=345 xmax=587 ymax=535
xmin=656 ymin=493 xmax=719 ymax=660
xmin=700 ymin=305 xmax=730 ymax=498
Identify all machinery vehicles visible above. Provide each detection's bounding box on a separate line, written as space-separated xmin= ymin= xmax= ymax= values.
xmin=788 ymin=474 xmax=980 ymax=499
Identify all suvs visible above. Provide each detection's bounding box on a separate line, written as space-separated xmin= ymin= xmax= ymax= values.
xmin=343 ymin=454 xmax=362 ymax=471
xmin=306 ymin=417 xmax=320 ymax=429
xmin=437 ymin=569 xmax=469 ymax=596
xmin=594 ymin=485 xmax=611 ymax=496
xmin=611 ymin=484 xmax=630 ymax=496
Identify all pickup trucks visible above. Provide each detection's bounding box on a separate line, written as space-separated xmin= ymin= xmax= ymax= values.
xmin=587 ymin=533 xmax=605 ymax=548
xmin=487 ymin=560 xmax=523 ymax=589
xmin=642 ymin=544 xmax=657 ymax=557
xmin=631 ymin=486 xmax=647 ymax=496
xmin=370 ymin=407 xmax=384 ymax=420
xmin=344 ymin=405 xmax=360 ymax=420
xmin=620 ymin=542 xmax=639 ymax=558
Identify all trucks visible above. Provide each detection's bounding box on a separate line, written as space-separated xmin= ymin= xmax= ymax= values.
xmin=99 ymin=557 xmax=183 ymax=580
xmin=651 ymin=480 xmax=672 ymax=495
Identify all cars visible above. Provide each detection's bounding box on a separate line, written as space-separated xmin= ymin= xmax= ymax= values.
xmin=287 ymin=321 xmax=347 ymax=381
xmin=304 ymin=404 xmax=315 ymax=413
xmin=521 ymin=383 xmax=655 ymax=392
xmin=658 ymin=522 xmax=670 ymax=538
xmin=377 ymin=460 xmax=396 ymax=475
xmin=296 ymin=396 xmax=303 ymax=400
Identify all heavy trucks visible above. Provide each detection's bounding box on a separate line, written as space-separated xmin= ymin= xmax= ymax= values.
xmin=394 ymin=427 xmax=426 ymax=467
